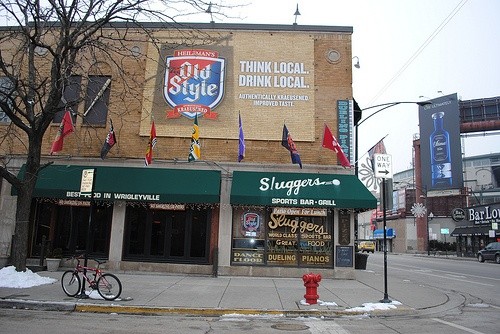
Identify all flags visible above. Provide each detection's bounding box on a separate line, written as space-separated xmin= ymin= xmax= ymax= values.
xmin=368 ymin=141 xmax=389 ymax=177
xmin=51 ymin=111 xmax=76 ymax=157
xmin=238 ymin=113 xmax=247 ymax=163
xmin=101 ymin=119 xmax=118 ymax=161
xmin=187 ymin=113 xmax=202 ymax=164
xmin=281 ymin=125 xmax=303 ymax=170
xmin=145 ymin=117 xmax=158 ymax=168
xmin=323 ymin=123 xmax=352 ymax=168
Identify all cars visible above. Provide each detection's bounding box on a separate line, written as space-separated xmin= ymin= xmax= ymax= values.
xmin=476 ymin=242 xmax=500 ymax=264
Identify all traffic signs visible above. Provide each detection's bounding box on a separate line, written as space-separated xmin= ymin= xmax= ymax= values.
xmin=372 ymin=153 xmax=393 ymax=180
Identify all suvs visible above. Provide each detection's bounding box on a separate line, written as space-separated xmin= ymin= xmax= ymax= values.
xmin=358 ymin=241 xmax=375 ymax=253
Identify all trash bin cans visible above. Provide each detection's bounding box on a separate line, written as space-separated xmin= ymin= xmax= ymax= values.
xmin=355 ymin=252 xmax=369 ymax=269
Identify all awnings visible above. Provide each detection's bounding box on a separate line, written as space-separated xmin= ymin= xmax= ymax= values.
xmin=230 ymin=170 xmax=379 ymax=211
xmin=12 ymin=162 xmax=223 ymax=205
xmin=370 ymin=228 xmax=396 ymax=240
xmin=451 ymin=228 xmax=492 ymax=238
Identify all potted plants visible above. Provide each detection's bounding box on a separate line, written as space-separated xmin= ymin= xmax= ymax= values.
xmin=45 ymin=246 xmax=63 ymax=270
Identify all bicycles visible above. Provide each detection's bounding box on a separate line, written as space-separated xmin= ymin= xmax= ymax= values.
xmin=61 ymin=255 xmax=122 ymax=302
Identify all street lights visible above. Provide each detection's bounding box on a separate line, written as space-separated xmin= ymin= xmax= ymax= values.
xmin=393 ymin=181 xmax=430 ymax=255
xmin=353 ymin=100 xmax=433 ymax=253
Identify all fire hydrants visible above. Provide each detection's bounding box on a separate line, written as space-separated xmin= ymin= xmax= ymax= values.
xmin=302 ymin=274 xmax=321 ymax=305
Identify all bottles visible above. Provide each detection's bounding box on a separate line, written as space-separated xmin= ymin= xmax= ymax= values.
xmin=430 ymin=112 xmax=453 ymax=189
